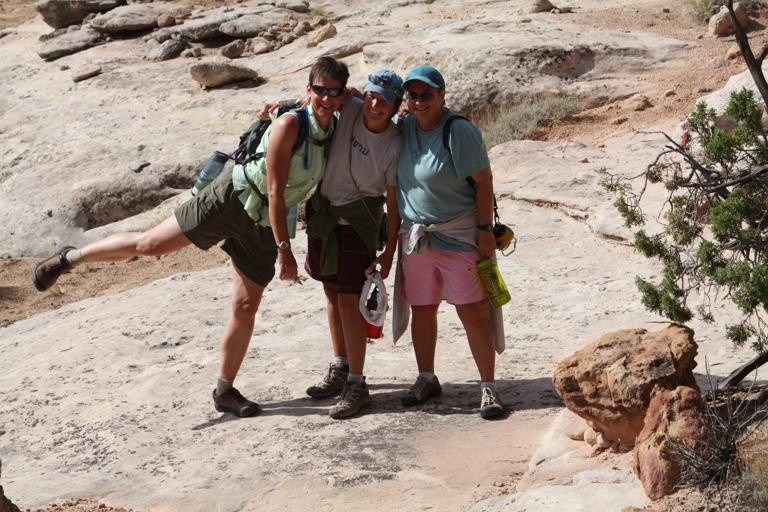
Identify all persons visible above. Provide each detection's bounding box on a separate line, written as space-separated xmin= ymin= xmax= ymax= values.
xmin=29 ymin=56 xmax=337 ymax=418
xmin=338 ymin=65 xmax=516 ymax=418
xmin=258 ymin=69 xmax=407 ymax=418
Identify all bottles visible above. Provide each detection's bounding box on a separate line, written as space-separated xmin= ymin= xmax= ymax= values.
xmin=478 ymin=258 xmax=510 ymax=307
xmin=288 ymin=201 xmax=299 ymax=239
xmin=192 ymin=151 xmax=228 ymax=199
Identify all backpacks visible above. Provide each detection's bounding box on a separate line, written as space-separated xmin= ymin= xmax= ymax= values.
xmin=233 ymin=99 xmax=303 ymax=166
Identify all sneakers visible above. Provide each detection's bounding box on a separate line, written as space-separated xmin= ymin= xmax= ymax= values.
xmin=479 ymin=382 xmax=504 ymax=419
xmin=32 ymin=245 xmax=81 ymax=291
xmin=213 ymin=387 xmax=259 ymax=418
xmin=306 ymin=365 xmax=350 ymax=399
xmin=329 ymin=380 xmax=371 ymax=420
xmin=402 ymin=373 xmax=442 ymax=407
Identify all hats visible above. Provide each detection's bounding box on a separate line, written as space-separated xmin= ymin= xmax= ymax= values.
xmin=400 ymin=65 xmax=445 ymax=89
xmin=364 ymin=70 xmax=405 ymax=103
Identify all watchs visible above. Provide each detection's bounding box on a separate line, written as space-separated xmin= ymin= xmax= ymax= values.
xmin=276 ymin=242 xmax=288 ymax=250
xmin=475 ymin=224 xmax=494 ymax=231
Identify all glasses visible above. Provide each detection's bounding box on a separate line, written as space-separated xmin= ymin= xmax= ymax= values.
xmin=310 ymin=84 xmax=347 ymax=98
xmin=405 ymin=91 xmax=442 ymax=100
